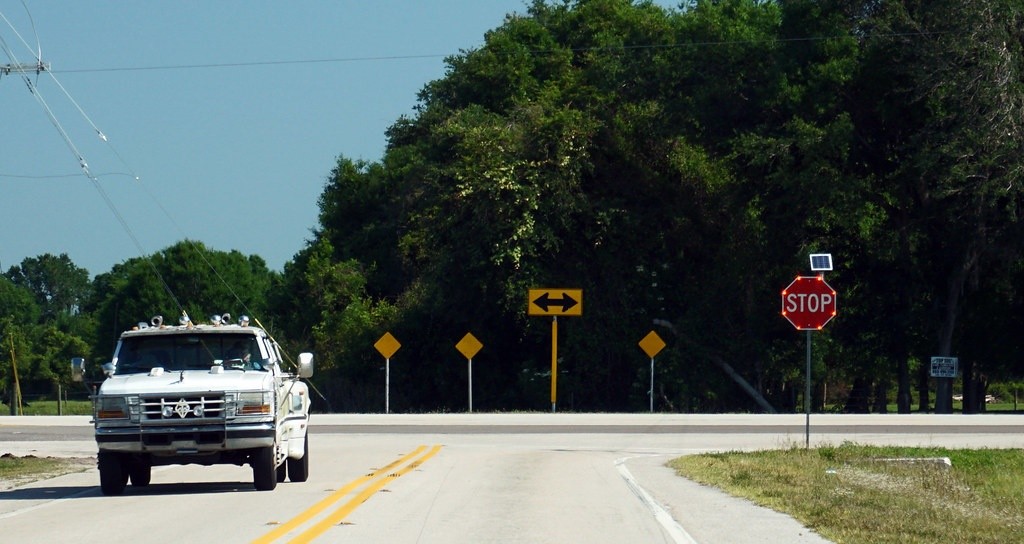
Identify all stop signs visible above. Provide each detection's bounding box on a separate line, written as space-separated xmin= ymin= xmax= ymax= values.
xmin=782 ymin=276 xmax=837 ymax=331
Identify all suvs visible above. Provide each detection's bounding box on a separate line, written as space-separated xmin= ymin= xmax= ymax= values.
xmin=94 ymin=310 xmax=313 ymax=496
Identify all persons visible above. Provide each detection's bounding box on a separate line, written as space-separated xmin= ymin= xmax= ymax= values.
xmin=220 ymin=339 xmax=262 ymax=371
